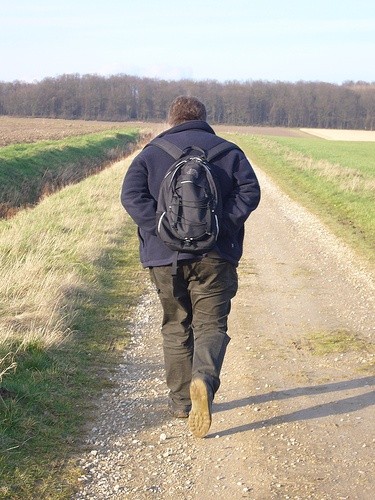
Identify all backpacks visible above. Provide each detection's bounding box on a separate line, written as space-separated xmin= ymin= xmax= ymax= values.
xmin=142 ymin=137 xmax=247 ymax=255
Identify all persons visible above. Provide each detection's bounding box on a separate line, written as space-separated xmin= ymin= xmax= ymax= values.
xmin=121 ymin=96 xmax=261 ymax=438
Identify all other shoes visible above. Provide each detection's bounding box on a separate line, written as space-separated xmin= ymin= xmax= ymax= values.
xmin=186 ymin=377 xmax=214 ymax=439
xmin=167 ymin=404 xmax=189 ymax=417
